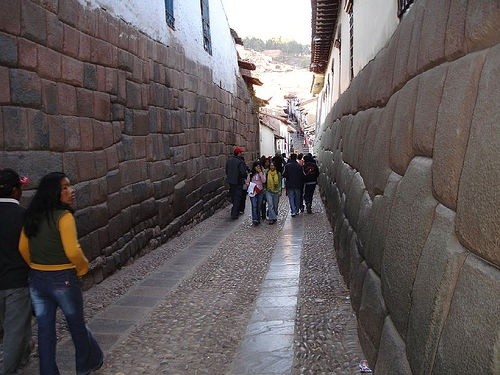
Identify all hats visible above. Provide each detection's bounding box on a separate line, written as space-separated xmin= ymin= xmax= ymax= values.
xmin=0 ymin=167 xmax=29 ymax=188
xmin=234 ymin=147 xmax=245 ymax=153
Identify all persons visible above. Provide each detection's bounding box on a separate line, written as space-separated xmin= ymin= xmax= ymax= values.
xmin=300 ymin=155 xmax=320 ymax=214
xmin=0 ymin=168 xmax=35 ymax=375
xmin=19 ymin=171 xmax=104 ymax=375
xmin=266 ymin=161 xmax=282 ymax=225
xmin=238 ymin=150 xmax=319 ymax=220
xmin=282 ymin=153 xmax=302 ymax=217
xmin=245 ymin=161 xmax=266 ymax=226
xmin=225 ymin=146 xmax=248 ymax=221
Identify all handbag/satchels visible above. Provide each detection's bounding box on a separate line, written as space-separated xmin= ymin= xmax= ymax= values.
xmin=227 ymin=190 xmax=242 ymax=203
xmin=243 ymin=184 xmax=247 ymax=190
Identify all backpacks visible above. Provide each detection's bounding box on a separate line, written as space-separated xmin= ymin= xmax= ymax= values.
xmin=304 ymin=161 xmax=317 ymax=182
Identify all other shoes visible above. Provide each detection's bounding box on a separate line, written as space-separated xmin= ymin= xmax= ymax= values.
xmin=252 ymin=220 xmax=260 ymax=226
xmin=307 ymin=208 xmax=312 ymax=214
xmin=28 ymin=337 xmax=35 ymax=352
xmin=291 ymin=212 xmax=298 ymax=217
xmin=269 ymin=219 xmax=277 ymax=225
xmin=301 ymin=205 xmax=304 ymax=212
xmin=85 ymin=359 xmax=104 ymax=375
